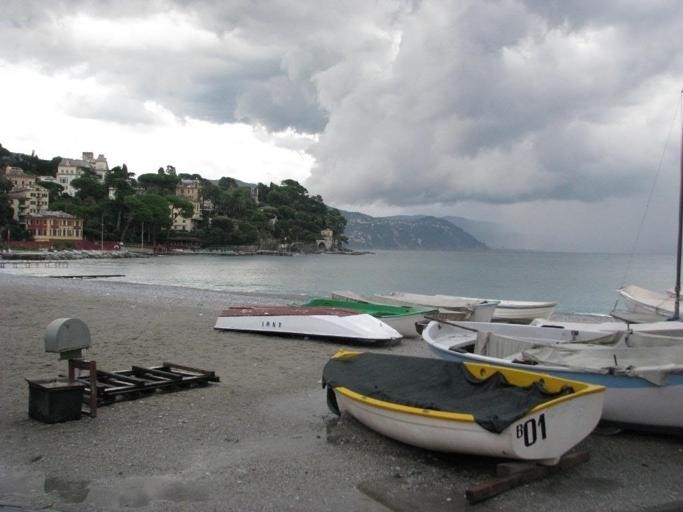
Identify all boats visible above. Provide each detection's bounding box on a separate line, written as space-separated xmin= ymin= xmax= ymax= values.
xmin=323 ymin=346 xmax=607 ymax=469
xmin=422 ymin=90 xmax=682 ymax=433
xmin=214 ymin=305 xmax=403 ymax=346
xmin=331 ymin=290 xmax=474 ymax=323
xmin=373 ymin=290 xmax=501 ymax=325
xmin=285 ymin=296 xmax=440 ymax=340
xmin=435 ymin=293 xmax=560 ymax=324
xmin=611 ymin=286 xmax=682 ymax=325
